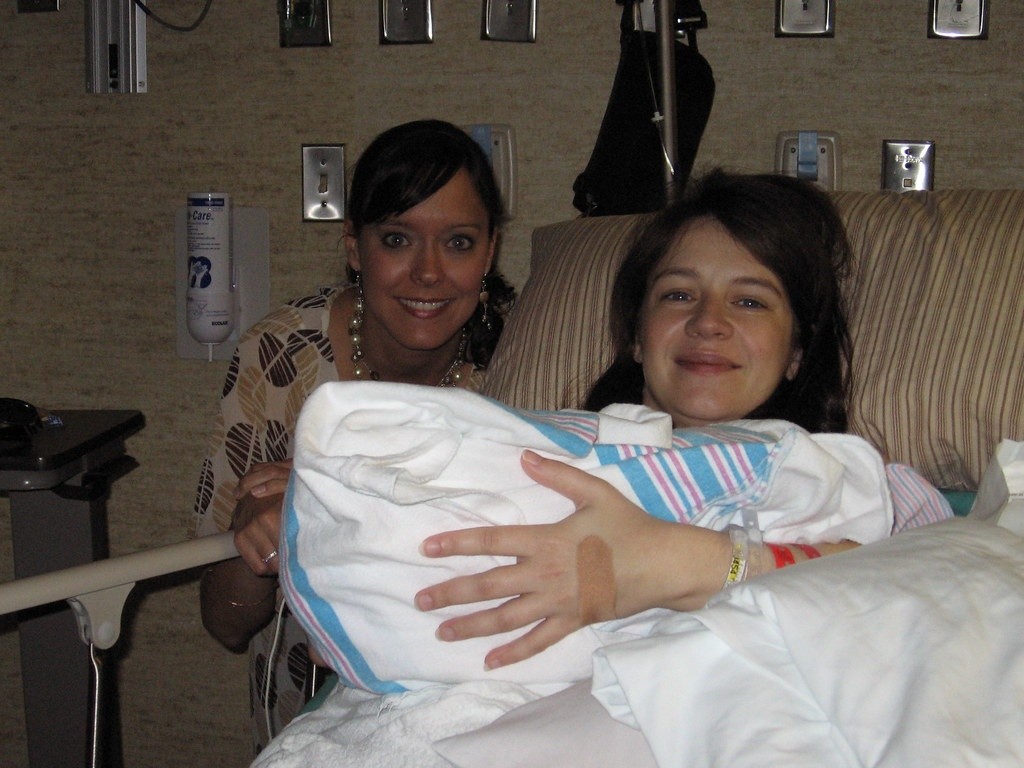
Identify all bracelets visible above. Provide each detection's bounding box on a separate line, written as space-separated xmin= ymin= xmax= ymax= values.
xmin=763 ymin=541 xmax=821 ymax=569
xmin=722 ymin=525 xmax=750 ymax=592
xmin=206 ymin=566 xmax=278 ymax=609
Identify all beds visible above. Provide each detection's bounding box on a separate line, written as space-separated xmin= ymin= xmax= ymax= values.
xmin=0 ymin=191 xmax=1024 ymax=768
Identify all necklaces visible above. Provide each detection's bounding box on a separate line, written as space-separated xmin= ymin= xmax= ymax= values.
xmin=349 ymin=290 xmax=469 ymax=387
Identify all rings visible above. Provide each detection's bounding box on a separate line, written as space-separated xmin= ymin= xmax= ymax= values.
xmin=263 ymin=551 xmax=277 ymax=562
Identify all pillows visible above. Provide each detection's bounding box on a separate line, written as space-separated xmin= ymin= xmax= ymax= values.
xmin=483 ymin=192 xmax=1024 ymax=491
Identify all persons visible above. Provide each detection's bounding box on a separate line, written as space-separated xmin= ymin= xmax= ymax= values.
xmin=194 ymin=120 xmax=516 ymax=650
xmin=308 ymin=173 xmax=956 ymax=669
